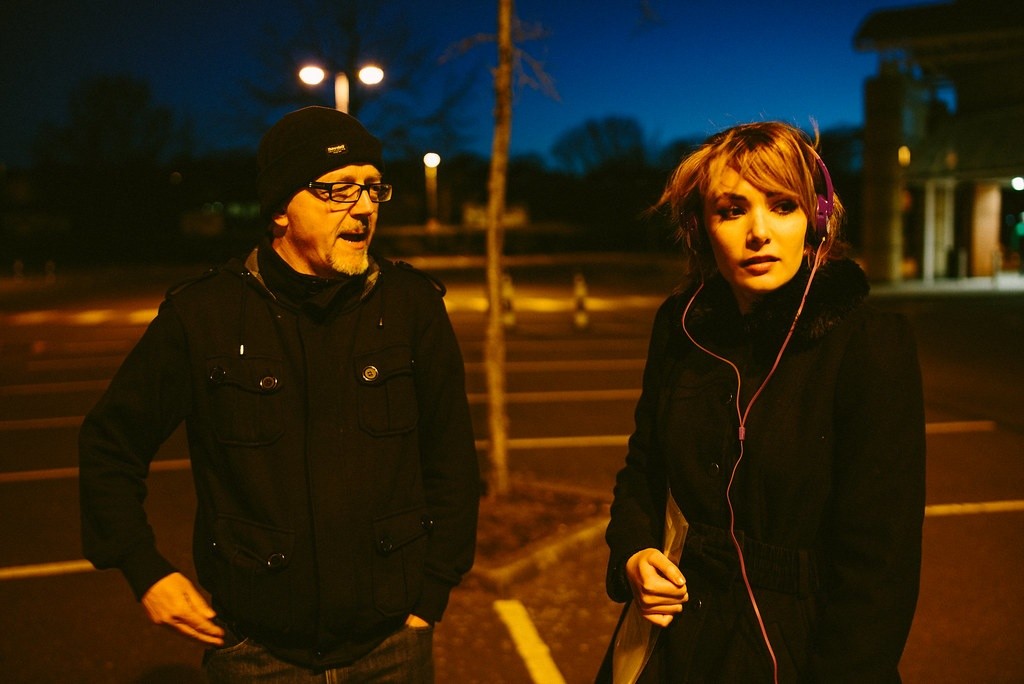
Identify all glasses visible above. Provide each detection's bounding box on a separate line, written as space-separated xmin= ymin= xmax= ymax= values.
xmin=303 ymin=180 xmax=393 ymax=203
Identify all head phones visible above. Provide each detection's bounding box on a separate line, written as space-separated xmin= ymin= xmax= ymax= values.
xmin=684 ymin=140 xmax=833 ymax=256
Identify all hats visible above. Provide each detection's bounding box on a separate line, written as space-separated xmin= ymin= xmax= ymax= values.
xmin=258 ymin=105 xmax=382 ymax=226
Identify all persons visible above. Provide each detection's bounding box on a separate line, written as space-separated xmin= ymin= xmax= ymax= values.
xmin=77 ymin=107 xmax=478 ymax=684
xmin=595 ymin=121 xmax=926 ymax=684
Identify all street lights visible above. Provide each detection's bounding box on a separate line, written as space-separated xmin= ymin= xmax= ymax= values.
xmin=299 ymin=62 xmax=385 ymax=119
